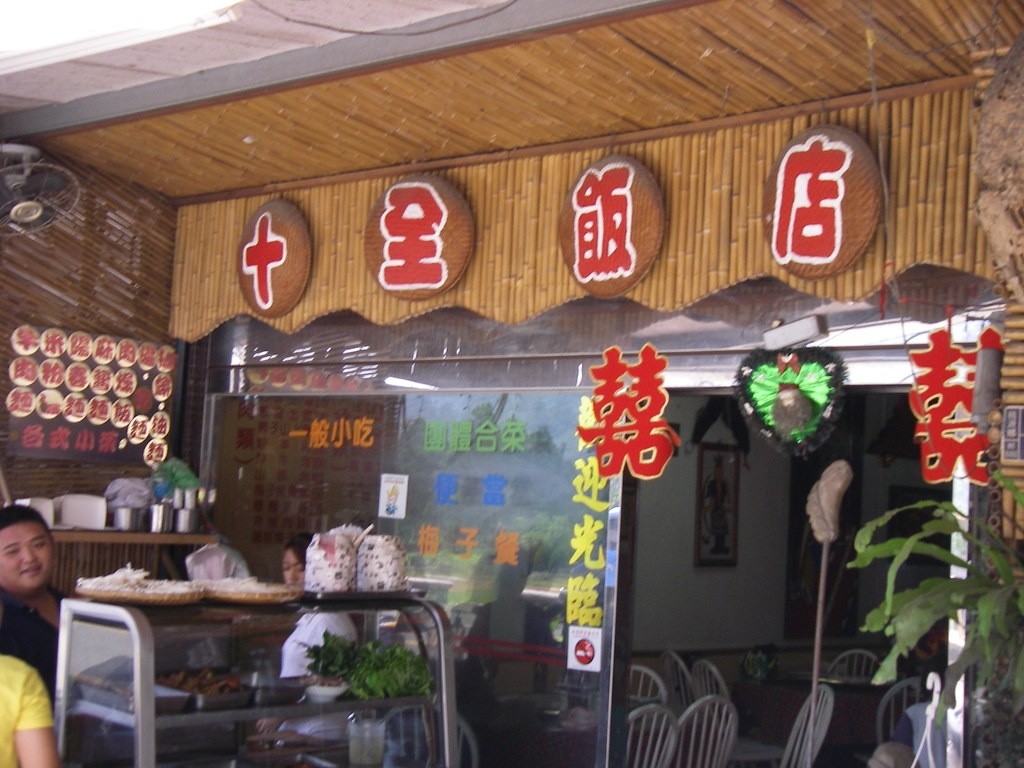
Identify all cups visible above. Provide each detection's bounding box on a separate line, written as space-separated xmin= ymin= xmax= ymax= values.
xmin=348 ymin=718 xmax=386 ymax=768
xmin=148 ymin=503 xmax=175 ymax=533
xmin=177 ymin=508 xmax=199 ymax=532
xmin=114 ymin=507 xmax=138 ymax=531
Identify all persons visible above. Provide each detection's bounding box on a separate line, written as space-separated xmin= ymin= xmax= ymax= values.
xmin=0 ymin=504 xmax=72 ymax=768
xmin=254 ymin=532 xmax=360 ymax=749
xmin=867 ymin=619 xmax=946 ymax=768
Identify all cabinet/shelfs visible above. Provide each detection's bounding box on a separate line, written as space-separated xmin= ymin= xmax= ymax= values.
xmin=53 ymin=591 xmax=460 ymax=768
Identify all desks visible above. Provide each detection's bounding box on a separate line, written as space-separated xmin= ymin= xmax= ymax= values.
xmin=739 ymin=669 xmax=908 ymax=768
xmin=458 ymin=690 xmax=712 ymax=768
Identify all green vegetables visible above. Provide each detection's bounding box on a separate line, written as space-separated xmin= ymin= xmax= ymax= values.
xmin=299 ymin=629 xmax=434 ymax=701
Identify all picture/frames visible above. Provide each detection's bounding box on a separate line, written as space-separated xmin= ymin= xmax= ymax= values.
xmin=695 ymin=443 xmax=740 ymax=567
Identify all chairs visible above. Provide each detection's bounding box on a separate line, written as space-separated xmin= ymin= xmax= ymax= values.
xmin=377 ymin=649 xmax=924 ymax=767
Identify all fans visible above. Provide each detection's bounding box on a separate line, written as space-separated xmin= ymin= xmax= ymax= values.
xmin=0 ymin=143 xmax=81 ymax=236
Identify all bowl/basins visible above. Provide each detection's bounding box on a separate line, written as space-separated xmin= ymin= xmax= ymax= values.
xmin=305 ymin=684 xmax=349 ymax=703
